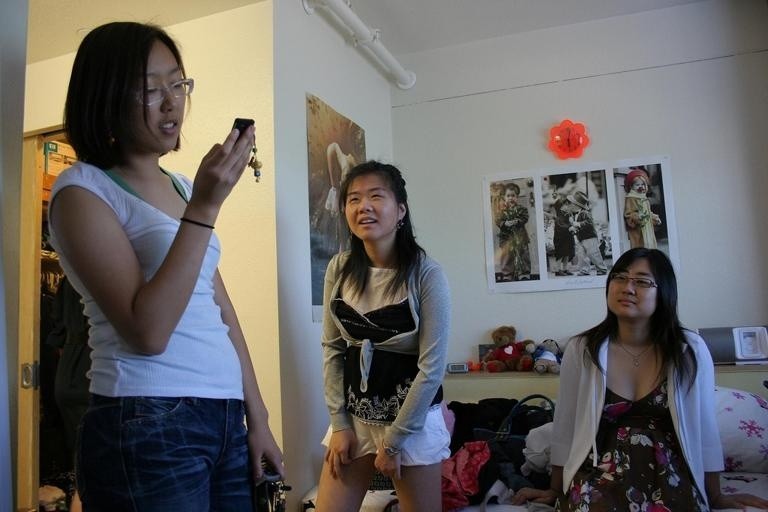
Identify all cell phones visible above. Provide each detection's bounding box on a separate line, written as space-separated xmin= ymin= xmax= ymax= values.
xmin=231 ymin=117 xmax=255 ymax=144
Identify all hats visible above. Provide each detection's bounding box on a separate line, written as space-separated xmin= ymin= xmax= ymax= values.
xmin=625 ymin=169 xmax=648 ymax=192
xmin=567 ymin=191 xmax=590 ymax=211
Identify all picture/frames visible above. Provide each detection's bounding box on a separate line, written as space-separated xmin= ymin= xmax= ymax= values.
xmin=480 ymin=153 xmax=681 ymax=295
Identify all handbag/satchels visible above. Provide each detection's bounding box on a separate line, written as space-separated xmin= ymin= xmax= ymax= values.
xmin=459 ymin=428 xmax=555 ymax=506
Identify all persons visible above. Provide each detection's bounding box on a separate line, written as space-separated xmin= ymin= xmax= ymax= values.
xmin=312 ymin=159 xmax=454 ymax=511
xmin=552 ymin=198 xmax=579 ymax=276
xmin=566 ymin=188 xmax=607 ymax=274
xmin=47 ymin=23 xmax=287 ymax=511
xmin=511 ymin=248 xmax=766 ymax=512
xmin=495 ymin=184 xmax=531 ymax=279
xmin=622 ymin=170 xmax=663 ymax=248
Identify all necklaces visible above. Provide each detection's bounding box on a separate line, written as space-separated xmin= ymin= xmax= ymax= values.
xmin=618 ymin=335 xmax=654 ymax=369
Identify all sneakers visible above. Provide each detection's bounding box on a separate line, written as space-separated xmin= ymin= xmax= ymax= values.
xmin=556 ymin=270 xmax=606 ymax=276
xmin=518 ymin=273 xmax=530 ymax=281
xmin=503 ymin=275 xmax=512 ymax=281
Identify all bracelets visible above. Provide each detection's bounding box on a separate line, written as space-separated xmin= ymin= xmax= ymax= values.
xmin=380 ymin=437 xmax=403 ymax=457
xmin=179 ymin=215 xmax=216 ymax=232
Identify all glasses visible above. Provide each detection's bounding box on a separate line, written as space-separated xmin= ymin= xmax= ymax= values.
xmin=611 ymin=272 xmax=658 ymax=289
xmin=130 ymin=79 xmax=195 ymax=106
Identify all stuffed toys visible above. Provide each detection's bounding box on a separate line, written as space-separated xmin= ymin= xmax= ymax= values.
xmin=526 ymin=337 xmax=564 ymax=373
xmin=480 ymin=326 xmax=536 ymax=371
